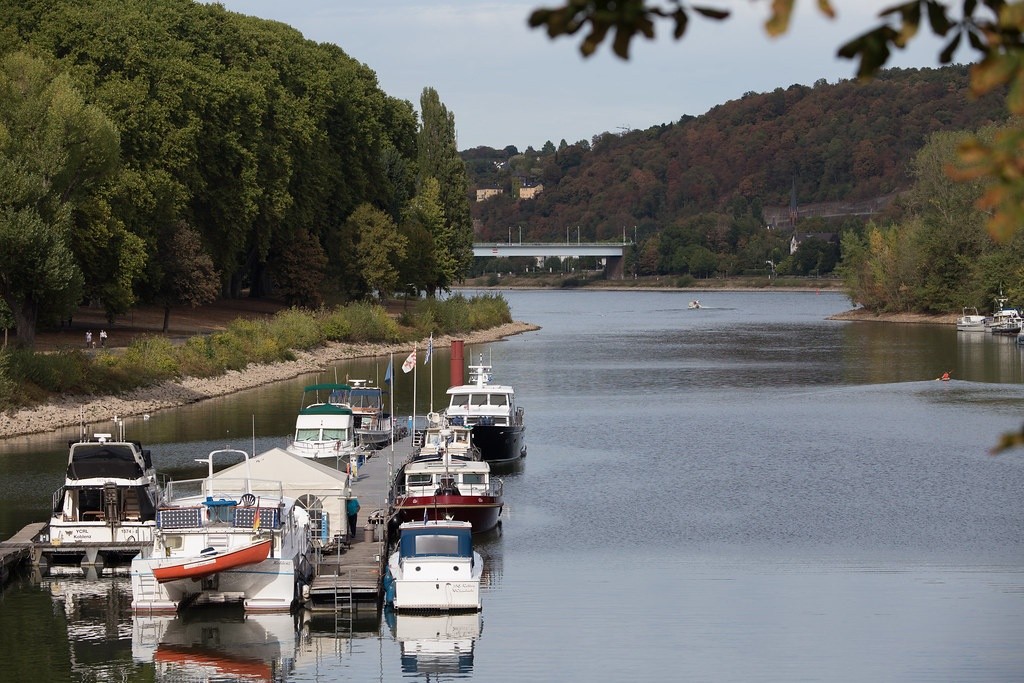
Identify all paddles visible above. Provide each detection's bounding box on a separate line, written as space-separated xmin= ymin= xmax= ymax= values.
xmin=935 ymin=369 xmax=955 ymax=381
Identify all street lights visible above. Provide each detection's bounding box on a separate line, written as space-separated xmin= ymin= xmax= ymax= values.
xmin=566 ymin=226 xmax=570 ymax=245
xmin=634 ymin=226 xmax=637 ymax=244
xmin=508 ymin=227 xmax=512 ymax=246
xmin=577 ymin=225 xmax=581 ymax=244
xmin=519 ymin=226 xmax=522 ymax=247
xmin=623 ymin=226 xmax=626 ymax=244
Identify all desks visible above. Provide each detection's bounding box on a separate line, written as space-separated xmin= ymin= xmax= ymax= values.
xmin=203 ymin=501 xmax=233 ymax=525
xmin=81 ymin=511 xmax=105 ymax=521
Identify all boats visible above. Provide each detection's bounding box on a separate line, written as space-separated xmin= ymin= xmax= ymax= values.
xmin=126 ymin=448 xmax=318 ymax=613
xmin=980 ymin=294 xmax=1023 ymax=334
xmin=283 ymin=382 xmax=372 ymax=475
xmin=126 ymin=608 xmax=316 ymax=683
xmin=151 ymin=647 xmax=276 ymax=683
xmin=956 ymin=307 xmax=987 ymax=332
xmin=390 ymin=408 xmax=505 ymax=535
xmin=687 ymin=299 xmax=702 ymax=309
xmin=37 ymin=408 xmax=171 ymax=578
xmin=381 ymin=609 xmax=484 ymax=682
xmin=151 ymin=537 xmax=273 ymax=586
xmin=428 ymin=349 xmax=529 ymax=463
xmin=47 ymin=553 xmax=138 ymax=681
xmin=322 ymin=366 xmax=402 ymax=449
xmin=382 ymin=493 xmax=485 ymax=613
xmin=1016 ymin=327 xmax=1024 ymax=346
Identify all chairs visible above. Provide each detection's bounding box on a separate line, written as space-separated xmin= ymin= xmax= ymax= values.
xmin=235 ymin=493 xmax=256 ymax=509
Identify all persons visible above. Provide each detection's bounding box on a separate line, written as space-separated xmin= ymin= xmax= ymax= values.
xmin=942 ymin=371 xmax=949 ymax=378
xmin=347 ymin=492 xmax=360 ymax=538
xmin=68 ymin=317 xmax=72 ymax=327
xmin=85 ymin=329 xmax=92 ymax=348
xmin=100 ymin=329 xmax=107 ymax=348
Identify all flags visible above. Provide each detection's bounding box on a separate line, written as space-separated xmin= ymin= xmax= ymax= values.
xmin=384 ymin=361 xmax=391 ymax=385
xmin=253 ymin=504 xmax=260 ymax=531
xmin=402 ymin=352 xmax=414 ymax=374
xmin=424 ymin=339 xmax=431 ymax=366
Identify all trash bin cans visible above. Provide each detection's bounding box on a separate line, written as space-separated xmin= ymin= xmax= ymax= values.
xmin=362 ymin=524 xmax=375 ymax=542
